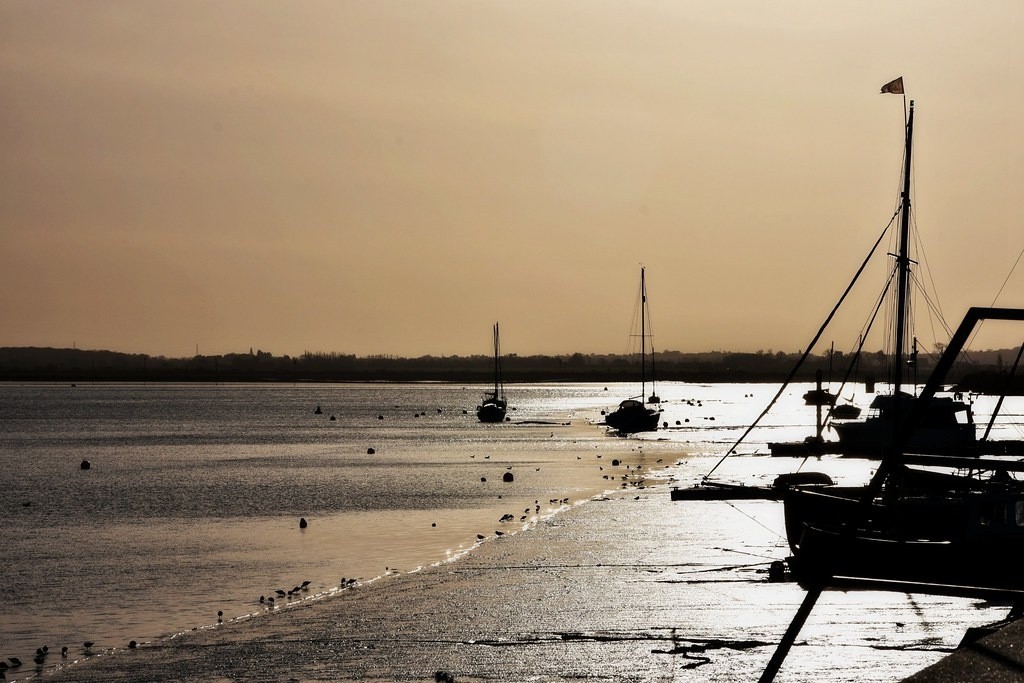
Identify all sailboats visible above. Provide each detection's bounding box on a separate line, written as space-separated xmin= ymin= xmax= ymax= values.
xmin=606 ymin=266 xmax=672 ymax=432
xmin=477 ymin=321 xmax=519 ymax=426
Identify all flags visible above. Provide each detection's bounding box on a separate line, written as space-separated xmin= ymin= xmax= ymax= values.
xmin=880 ymin=76 xmax=904 ymax=94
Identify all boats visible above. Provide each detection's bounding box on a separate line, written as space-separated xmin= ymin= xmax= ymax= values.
xmin=668 ymin=99 xmax=1024 ymax=602
